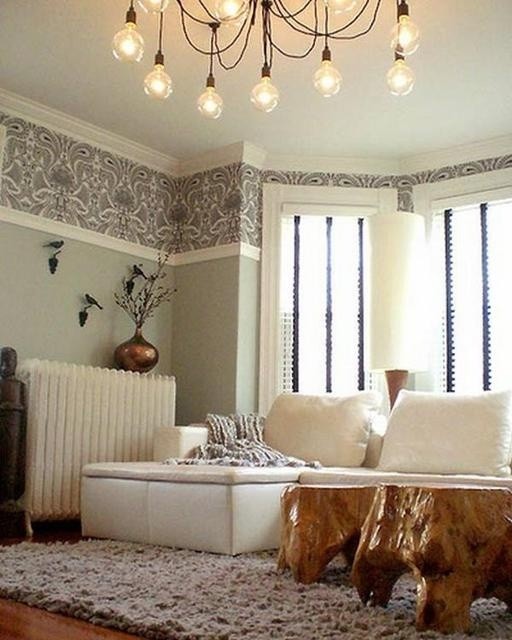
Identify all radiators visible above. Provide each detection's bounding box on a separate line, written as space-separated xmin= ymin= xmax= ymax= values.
xmin=2 ymin=357 xmax=179 ymax=538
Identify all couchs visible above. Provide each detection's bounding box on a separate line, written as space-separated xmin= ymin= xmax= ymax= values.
xmin=75 ymin=390 xmax=512 ymax=561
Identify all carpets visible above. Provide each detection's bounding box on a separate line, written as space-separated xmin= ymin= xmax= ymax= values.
xmin=1 ymin=538 xmax=511 ymax=640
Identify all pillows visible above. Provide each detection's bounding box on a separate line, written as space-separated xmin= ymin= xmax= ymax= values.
xmin=259 ymin=389 xmax=510 ymax=477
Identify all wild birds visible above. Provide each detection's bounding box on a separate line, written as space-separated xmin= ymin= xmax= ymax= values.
xmin=133 ymin=264 xmax=148 ymax=279
xmin=85 ymin=294 xmax=103 ymax=310
xmin=43 ymin=240 xmax=64 ymax=248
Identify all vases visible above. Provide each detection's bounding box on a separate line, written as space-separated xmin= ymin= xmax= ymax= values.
xmin=114 ymin=327 xmax=160 ymax=373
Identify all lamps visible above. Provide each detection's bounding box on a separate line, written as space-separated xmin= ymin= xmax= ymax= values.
xmin=113 ymin=1 xmax=422 ymax=120
